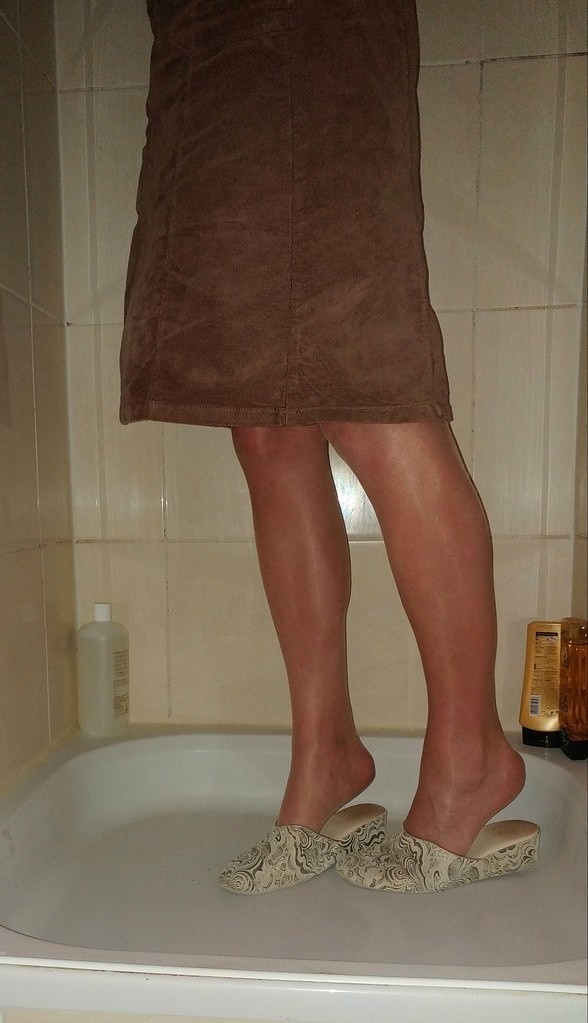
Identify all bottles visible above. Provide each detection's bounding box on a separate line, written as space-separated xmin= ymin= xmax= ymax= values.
xmin=75 ymin=602 xmax=131 ymax=739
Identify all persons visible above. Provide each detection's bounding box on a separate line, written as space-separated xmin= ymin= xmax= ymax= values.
xmin=119 ymin=0 xmax=539 ymax=892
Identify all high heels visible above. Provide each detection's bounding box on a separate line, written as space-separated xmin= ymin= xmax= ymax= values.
xmin=335 ymin=819 xmax=541 ymax=894
xmin=217 ymin=802 xmax=389 ymax=896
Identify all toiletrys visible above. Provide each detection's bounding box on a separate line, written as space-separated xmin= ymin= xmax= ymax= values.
xmin=518 ymin=616 xmax=588 ymax=762
xmin=74 ymin=601 xmax=131 ymax=739
